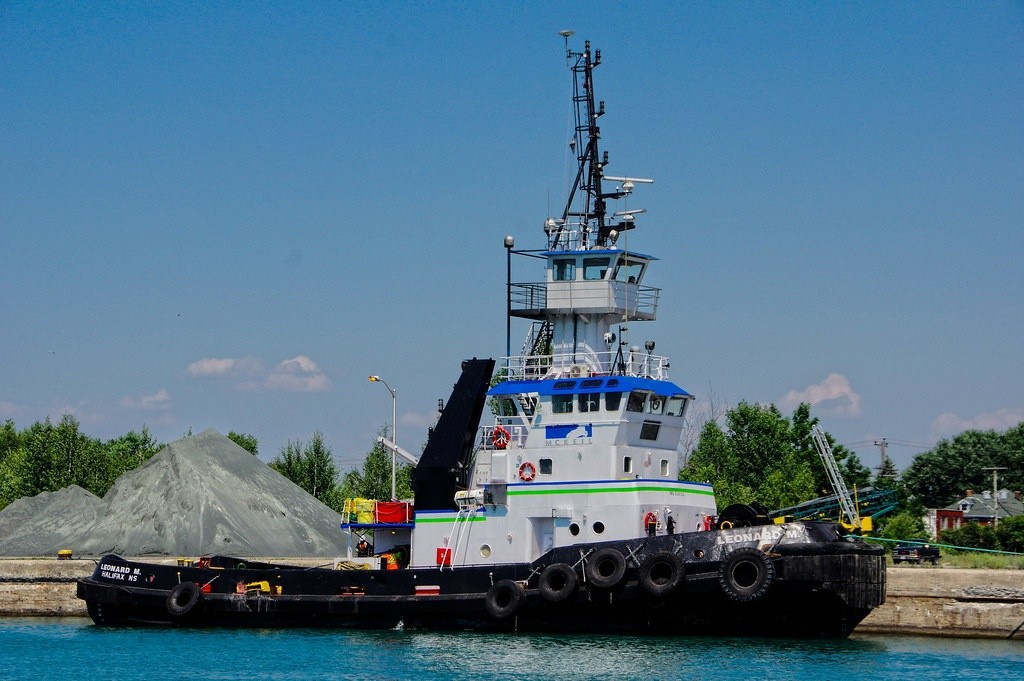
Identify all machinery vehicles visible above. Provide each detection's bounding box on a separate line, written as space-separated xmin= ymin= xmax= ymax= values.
xmin=773 ymin=424 xmax=872 ymax=545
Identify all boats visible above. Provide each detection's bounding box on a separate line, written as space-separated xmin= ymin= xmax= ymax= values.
xmin=75 ymin=29 xmax=886 ymax=642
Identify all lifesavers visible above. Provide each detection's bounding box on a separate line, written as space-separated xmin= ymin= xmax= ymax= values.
xmin=166 ymin=581 xmax=202 ymax=613
xmin=719 ymin=547 xmax=775 ymax=603
xmin=637 ymin=551 xmax=685 ymax=594
xmin=538 ymin=563 xmax=578 ymax=601
xmin=585 ymin=548 xmax=627 ymax=588
xmin=519 ymin=462 xmax=536 ymax=481
xmin=484 ymin=579 xmax=522 ymax=620
xmin=492 ymin=428 xmax=512 ymax=449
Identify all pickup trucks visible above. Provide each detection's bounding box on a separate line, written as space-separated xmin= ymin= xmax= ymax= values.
xmin=893 ymin=539 xmax=940 ymax=565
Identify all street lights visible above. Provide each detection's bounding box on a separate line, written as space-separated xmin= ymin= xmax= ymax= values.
xmin=369 ymin=376 xmax=396 ymax=498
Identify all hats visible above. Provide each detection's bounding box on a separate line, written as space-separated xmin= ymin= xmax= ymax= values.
xmin=359 ymin=535 xmax=365 ymax=540
